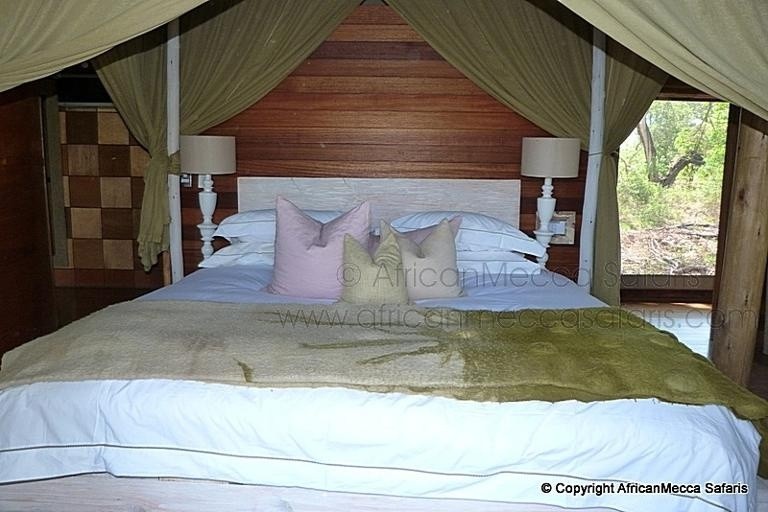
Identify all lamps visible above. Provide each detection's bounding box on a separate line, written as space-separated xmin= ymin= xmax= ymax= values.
xmin=175 ymin=132 xmax=237 ymax=266
xmin=515 ymin=135 xmax=581 ymax=267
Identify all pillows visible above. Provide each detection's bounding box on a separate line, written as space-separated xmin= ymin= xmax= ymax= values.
xmin=213 ymin=201 xmax=548 ymax=308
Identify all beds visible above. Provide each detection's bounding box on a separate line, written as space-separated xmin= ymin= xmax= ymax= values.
xmin=0 ymin=176 xmax=763 ymax=506
